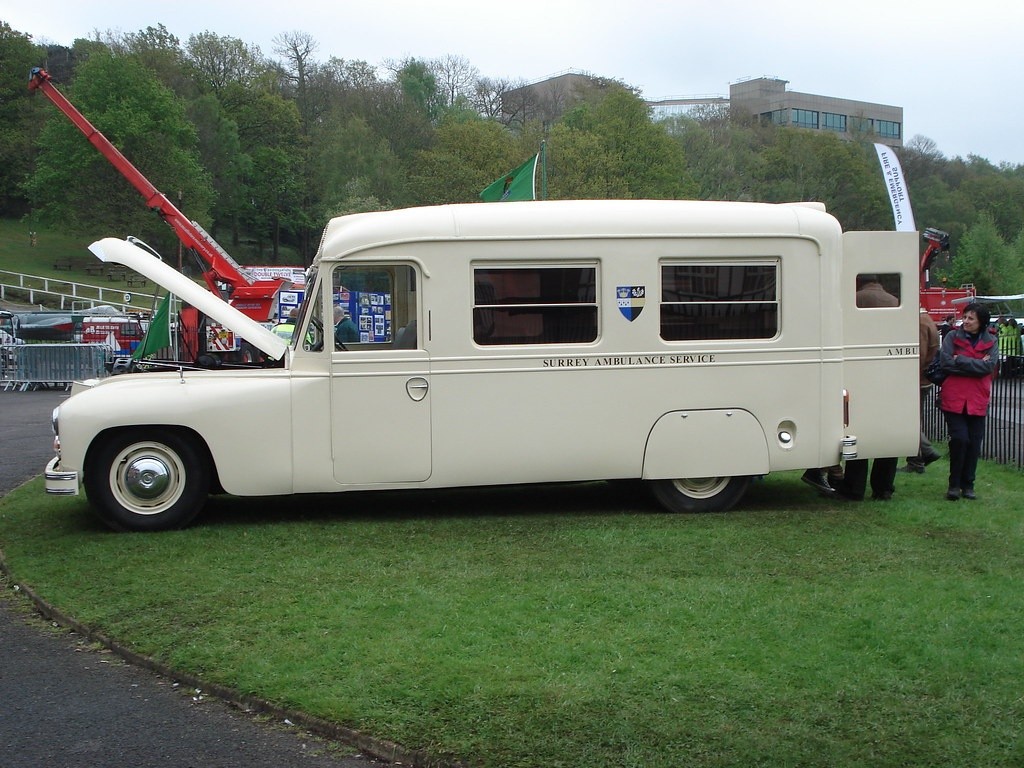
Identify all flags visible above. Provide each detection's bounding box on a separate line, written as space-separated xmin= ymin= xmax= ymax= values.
xmin=130 ymin=289 xmax=173 ymax=360
xmin=479 ymin=150 xmax=541 ymax=202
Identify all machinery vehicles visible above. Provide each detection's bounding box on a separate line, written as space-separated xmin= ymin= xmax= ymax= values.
xmin=27 ymin=65 xmax=323 ymax=373
xmin=918 ymin=226 xmax=977 ymax=329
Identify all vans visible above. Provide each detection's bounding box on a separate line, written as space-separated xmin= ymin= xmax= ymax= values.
xmin=82 ymin=316 xmax=153 ymax=357
xmin=43 ymin=199 xmax=921 ymax=534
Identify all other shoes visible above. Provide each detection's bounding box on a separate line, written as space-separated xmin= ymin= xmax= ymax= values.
xmin=901 ymin=463 xmax=925 ymax=474
xmin=947 ymin=487 xmax=960 ymax=501
xmin=801 ymin=469 xmax=835 ymax=492
xmin=836 ymin=475 xmax=864 ymax=501
xmin=962 ymin=486 xmax=977 ymax=500
xmin=872 ymin=490 xmax=891 ymax=500
xmin=924 ymin=451 xmax=941 ymax=465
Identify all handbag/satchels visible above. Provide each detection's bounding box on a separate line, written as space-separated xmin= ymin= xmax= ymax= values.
xmin=924 ymin=331 xmax=957 ymax=387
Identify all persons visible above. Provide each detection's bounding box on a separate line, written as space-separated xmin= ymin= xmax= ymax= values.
xmin=798 ymin=272 xmax=1024 ymax=505
xmin=333 ymin=305 xmax=360 ymax=343
xmin=270 ymin=308 xmax=312 ymax=351
xmin=940 ymin=302 xmax=1000 ymax=502
